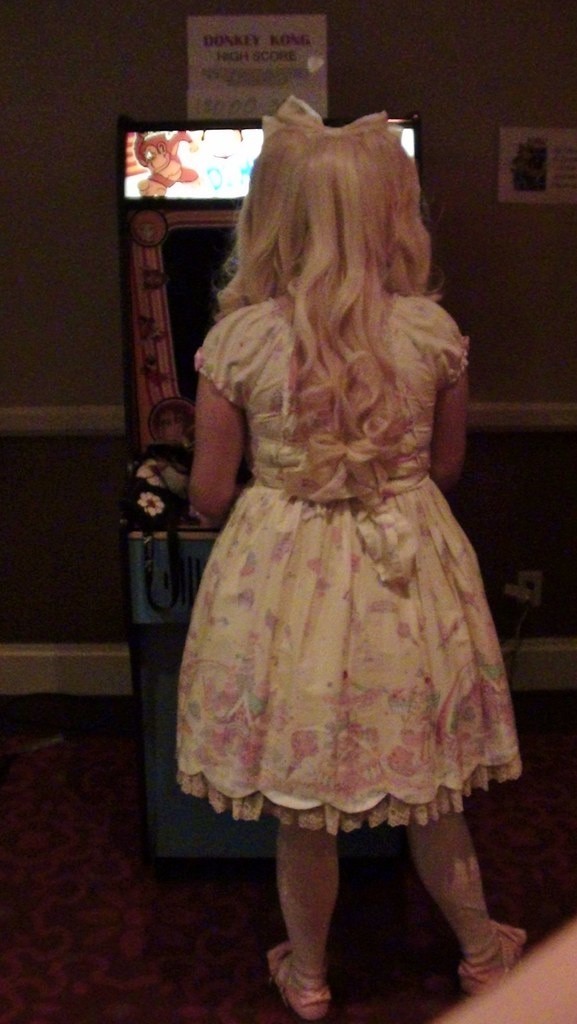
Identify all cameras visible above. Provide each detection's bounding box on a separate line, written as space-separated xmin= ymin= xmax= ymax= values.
xmin=118 ymin=442 xmax=252 ymax=533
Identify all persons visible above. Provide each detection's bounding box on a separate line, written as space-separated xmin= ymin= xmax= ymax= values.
xmin=176 ymin=97 xmax=527 ymax=1024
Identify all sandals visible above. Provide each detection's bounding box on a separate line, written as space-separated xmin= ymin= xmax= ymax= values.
xmin=267 ymin=936 xmax=331 ymax=1019
xmin=460 ymin=919 xmax=527 ymax=998
